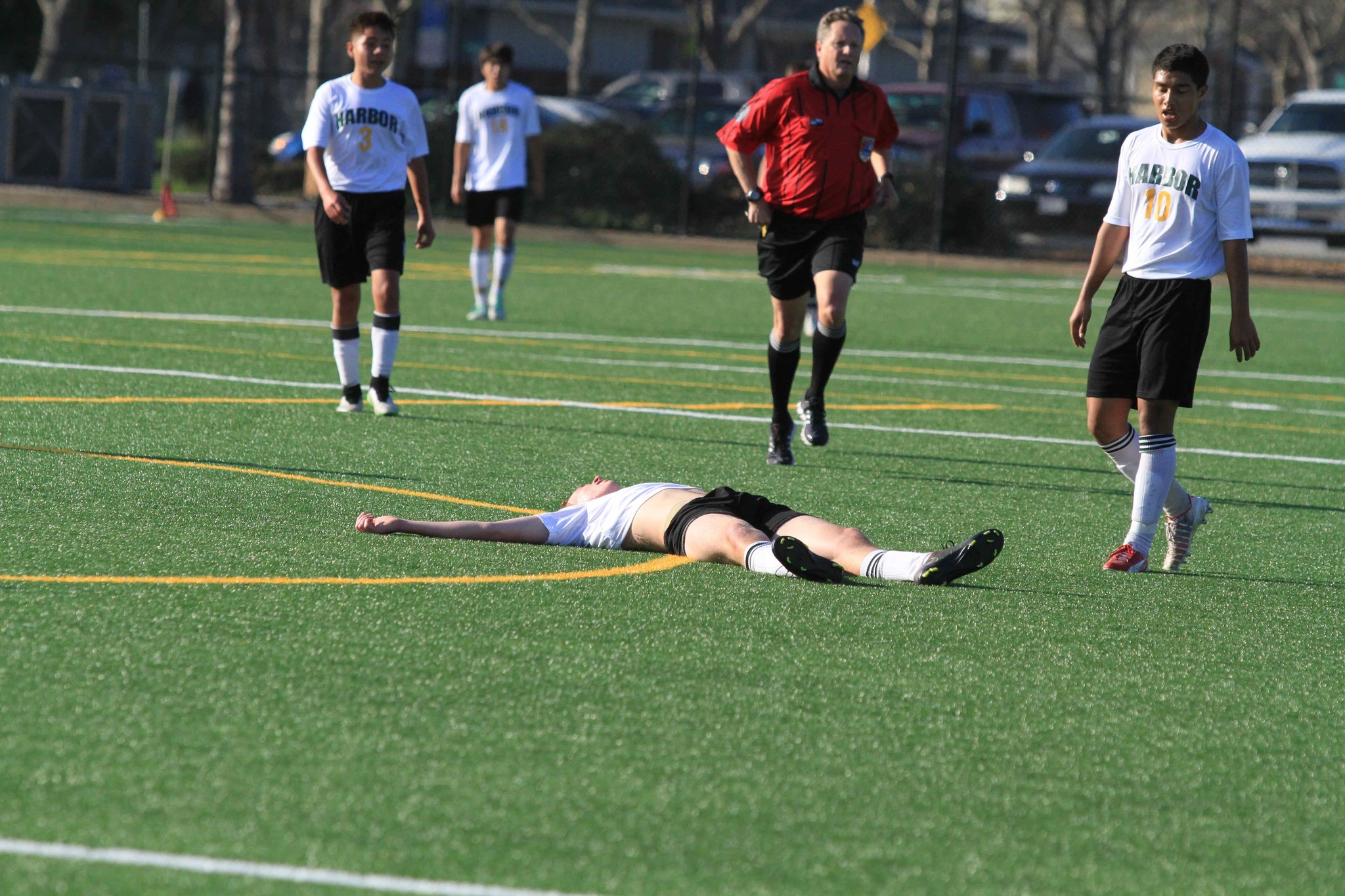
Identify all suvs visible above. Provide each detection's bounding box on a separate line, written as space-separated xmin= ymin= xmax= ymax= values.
xmin=1233 ymin=88 xmax=1345 ymax=247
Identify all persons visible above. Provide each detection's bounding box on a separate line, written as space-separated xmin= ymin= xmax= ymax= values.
xmin=450 ymin=40 xmax=545 ymax=322
xmin=712 ymin=6 xmax=900 ymax=461
xmin=1066 ymin=40 xmax=1262 ymax=574
xmin=299 ymin=11 xmax=439 ymax=417
xmin=352 ymin=474 xmax=1010 ymax=587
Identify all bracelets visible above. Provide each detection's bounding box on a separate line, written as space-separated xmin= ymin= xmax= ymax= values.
xmin=879 ymin=171 xmax=895 ymax=186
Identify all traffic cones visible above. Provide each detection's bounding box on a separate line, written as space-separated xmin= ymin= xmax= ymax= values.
xmin=161 ymin=181 xmax=171 ymax=211
xmin=164 ymin=189 xmax=178 ymax=217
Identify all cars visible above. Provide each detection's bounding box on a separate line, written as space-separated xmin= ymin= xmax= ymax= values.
xmin=996 ymin=116 xmax=1160 ymax=232
xmin=530 ymin=71 xmax=1097 ymax=201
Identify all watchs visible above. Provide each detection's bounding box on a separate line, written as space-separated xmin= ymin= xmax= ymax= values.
xmin=743 ymin=187 xmax=765 ymax=203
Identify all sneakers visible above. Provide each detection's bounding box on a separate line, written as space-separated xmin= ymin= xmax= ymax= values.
xmin=1163 ymin=494 xmax=1213 ymax=570
xmin=772 ymin=536 xmax=845 ymax=585
xmin=1102 ymin=531 xmax=1153 ymax=573
xmin=766 ymin=413 xmax=796 ymax=466
xmin=466 ymin=289 xmax=506 ymax=320
xmin=336 ymin=385 xmax=362 ymax=412
xmin=914 ymin=529 xmax=1004 ymax=586
xmin=795 ymin=387 xmax=829 ymax=447
xmin=366 ymin=378 xmax=397 ymax=416
